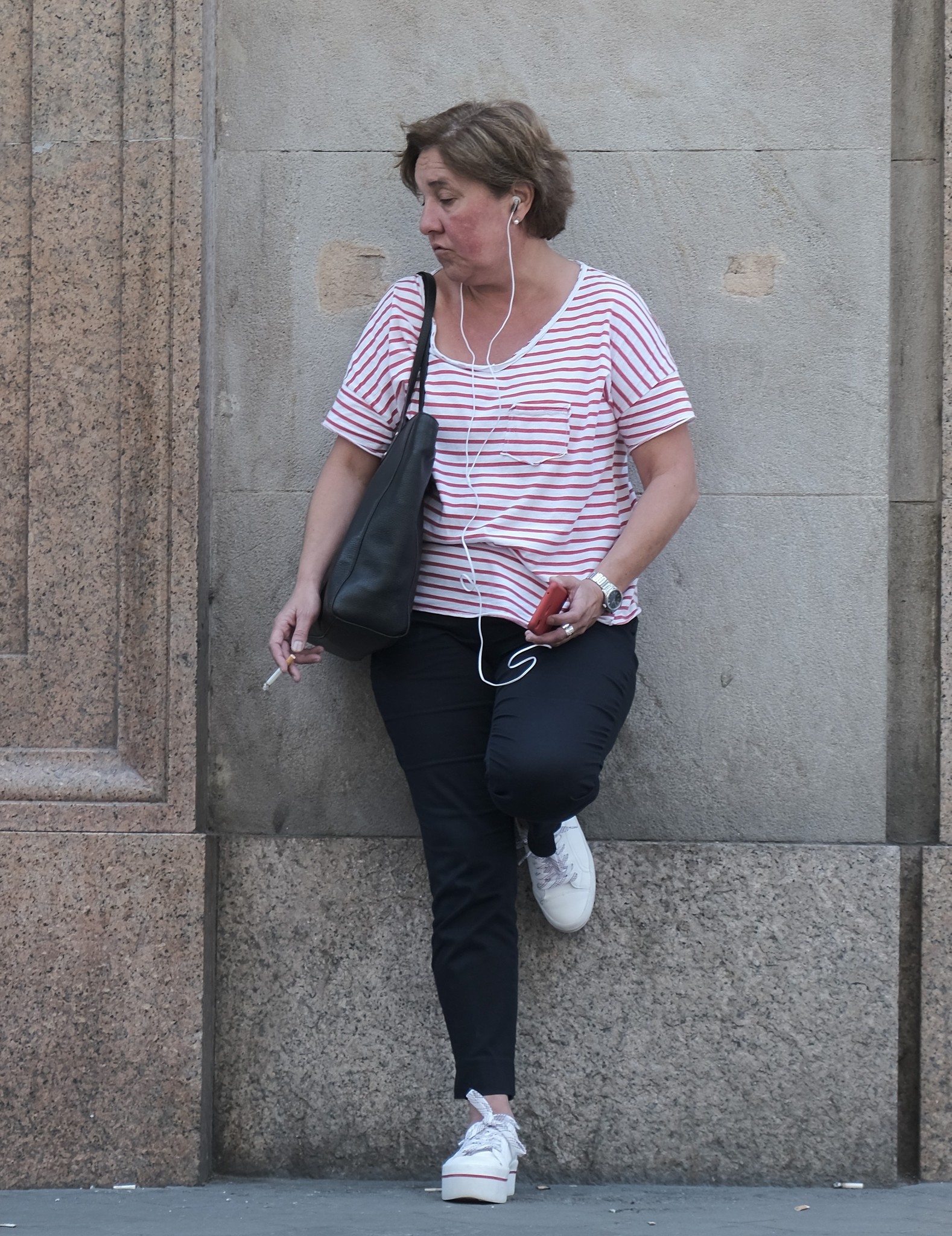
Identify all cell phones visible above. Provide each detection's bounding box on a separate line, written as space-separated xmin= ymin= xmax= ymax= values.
xmin=528 ymin=581 xmax=567 ymax=635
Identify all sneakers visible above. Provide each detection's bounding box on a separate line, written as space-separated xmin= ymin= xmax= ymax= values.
xmin=442 ymin=1089 xmax=526 ymax=1204
xmin=516 ymin=811 xmax=596 ymax=933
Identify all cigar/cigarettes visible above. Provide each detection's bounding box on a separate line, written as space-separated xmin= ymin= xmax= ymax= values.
xmin=261 ymin=653 xmax=296 ymax=689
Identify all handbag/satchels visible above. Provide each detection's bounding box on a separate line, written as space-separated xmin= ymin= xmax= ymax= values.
xmin=307 ymin=271 xmax=437 ymax=661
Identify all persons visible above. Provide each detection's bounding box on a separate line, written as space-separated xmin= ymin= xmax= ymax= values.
xmin=267 ymin=104 xmax=697 ymax=1204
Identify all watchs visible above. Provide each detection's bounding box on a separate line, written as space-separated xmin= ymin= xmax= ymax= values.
xmin=586 ymin=572 xmax=623 ymax=615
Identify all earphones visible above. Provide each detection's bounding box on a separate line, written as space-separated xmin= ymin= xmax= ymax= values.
xmin=511 ymin=196 xmax=522 ymax=212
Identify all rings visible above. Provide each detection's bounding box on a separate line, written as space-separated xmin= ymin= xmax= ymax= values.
xmin=562 ymin=622 xmax=574 ymax=637
